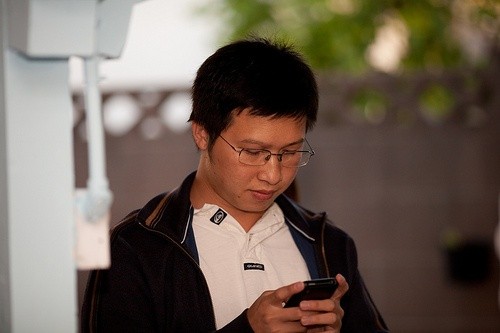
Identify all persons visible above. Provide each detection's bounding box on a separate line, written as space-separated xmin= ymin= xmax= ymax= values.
xmin=78 ymin=32 xmax=388 ymax=332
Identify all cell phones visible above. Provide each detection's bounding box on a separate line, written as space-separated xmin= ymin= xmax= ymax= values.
xmin=286 ymin=278 xmax=338 ymax=308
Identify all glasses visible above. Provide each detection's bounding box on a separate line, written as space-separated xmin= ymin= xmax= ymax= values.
xmin=220 ymin=135 xmax=315 ymax=168
